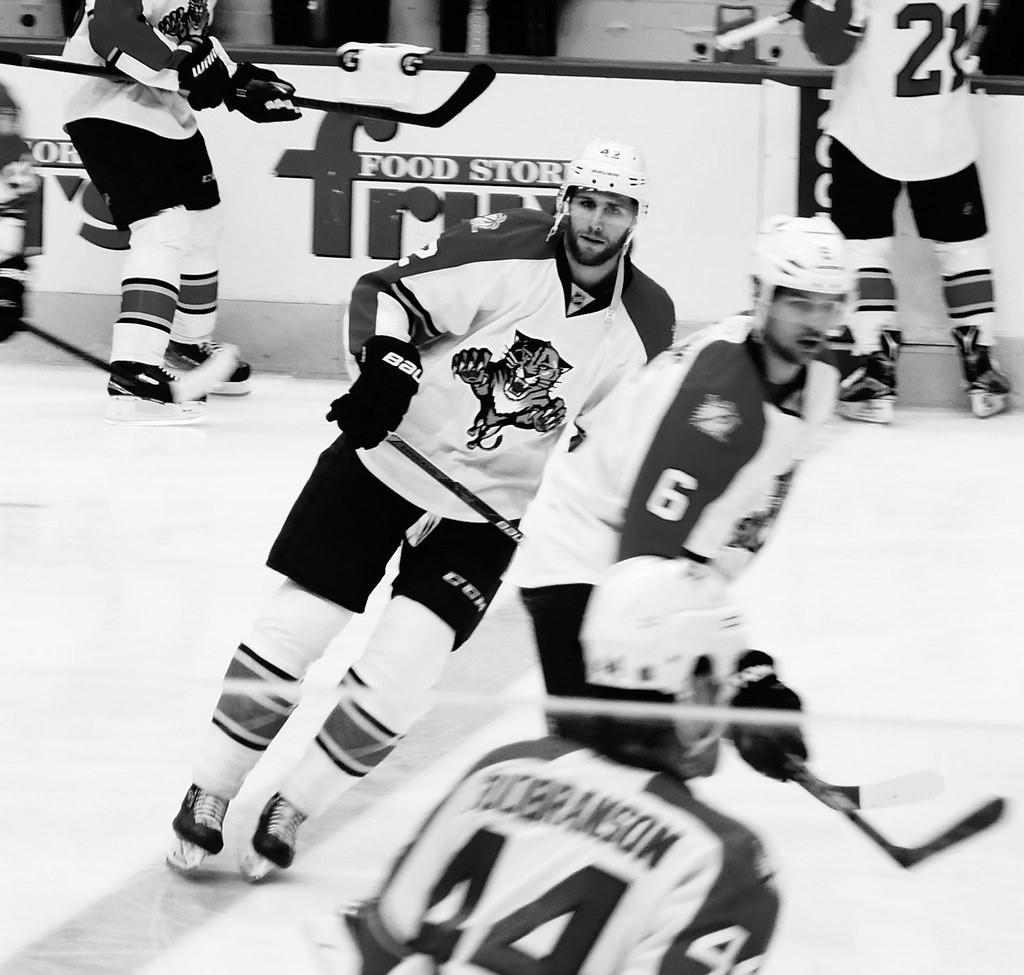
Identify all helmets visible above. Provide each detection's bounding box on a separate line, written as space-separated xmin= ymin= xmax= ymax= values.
xmin=580 ymin=555 xmax=744 ymax=696
xmin=750 ymin=213 xmax=855 ymax=294
xmin=556 ymin=137 xmax=648 ymax=250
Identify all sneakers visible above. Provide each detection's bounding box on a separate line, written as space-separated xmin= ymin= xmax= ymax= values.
xmin=105 ymin=361 xmax=208 ymax=426
xmin=834 ymin=350 xmax=897 ymax=424
xmin=160 ymin=340 xmax=252 ymax=396
xmin=951 ymin=325 xmax=1010 ymax=418
xmin=239 ymin=793 xmax=306 ymax=882
xmin=167 ymin=781 xmax=228 ymax=872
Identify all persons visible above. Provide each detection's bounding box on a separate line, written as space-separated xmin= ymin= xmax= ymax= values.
xmin=270 ymin=0 xmax=570 ymax=58
xmin=513 ymin=216 xmax=861 ymax=774
xmin=2 ymin=80 xmax=44 ymax=341
xmin=57 ymin=0 xmax=307 ymax=398
xmin=171 ymin=138 xmax=676 ymax=888
xmin=790 ymin=1 xmax=1010 ymax=423
xmin=346 ymin=554 xmax=780 ymax=975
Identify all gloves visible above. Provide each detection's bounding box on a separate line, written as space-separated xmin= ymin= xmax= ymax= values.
xmin=177 ymin=35 xmax=237 ymax=111
xmin=325 ymin=335 xmax=422 ymax=451
xmin=224 ymin=62 xmax=302 ymax=122
xmin=790 ymin=0 xmax=811 ymax=23
xmin=731 ymin=651 xmax=807 ymax=781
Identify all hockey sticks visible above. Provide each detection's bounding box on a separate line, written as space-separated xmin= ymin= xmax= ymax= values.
xmin=764 ymin=739 xmax=1006 ymax=870
xmin=0 ymin=49 xmax=496 ymax=129
xmin=20 ymin=323 xmax=242 ymax=406
xmin=386 ymin=433 xmax=942 ymax=813
xmin=707 ymin=0 xmax=826 ymax=55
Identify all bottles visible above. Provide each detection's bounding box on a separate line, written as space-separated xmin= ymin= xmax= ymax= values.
xmin=465 ymin=0 xmax=489 ymax=54
